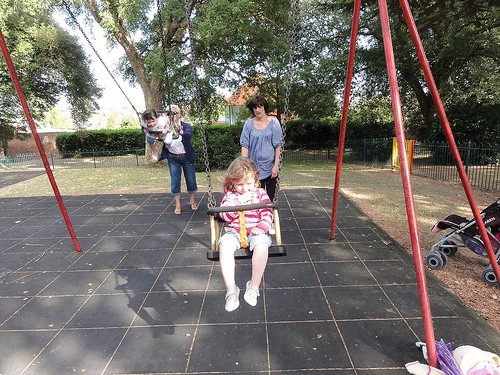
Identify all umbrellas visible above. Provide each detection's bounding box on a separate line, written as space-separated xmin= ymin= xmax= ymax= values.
xmin=416 ymin=339 xmax=464 ymax=375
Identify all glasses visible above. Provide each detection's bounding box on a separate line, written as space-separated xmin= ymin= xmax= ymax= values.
xmin=167 ymin=112 xmax=177 ymax=116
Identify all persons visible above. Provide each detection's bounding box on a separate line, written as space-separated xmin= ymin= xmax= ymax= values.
xmin=218 ymin=156 xmax=274 ymax=312
xmin=139 ymin=105 xmax=199 ymax=215
xmin=240 ymin=97 xmax=282 ymax=235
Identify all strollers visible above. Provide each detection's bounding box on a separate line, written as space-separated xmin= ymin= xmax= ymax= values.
xmin=425 ymin=197 xmax=500 ymax=285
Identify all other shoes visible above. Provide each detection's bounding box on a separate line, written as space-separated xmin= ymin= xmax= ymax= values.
xmin=224 ymin=285 xmax=241 ymax=312
xmin=174 ymin=207 xmax=181 ymax=215
xmin=244 ymin=281 xmax=260 ymax=307
xmin=189 ymin=201 xmax=199 ymax=210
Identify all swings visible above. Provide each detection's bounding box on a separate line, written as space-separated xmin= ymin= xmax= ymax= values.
xmin=183 ymin=0 xmax=301 ymax=260
xmin=62 ymin=0 xmax=171 ymax=145
